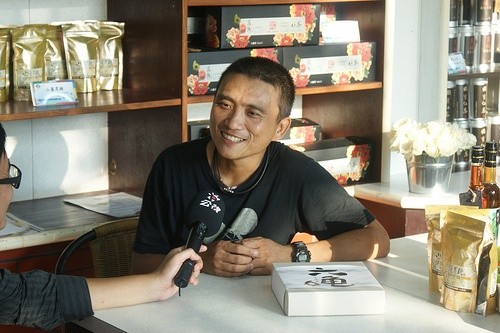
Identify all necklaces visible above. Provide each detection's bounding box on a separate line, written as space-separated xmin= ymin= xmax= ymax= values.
xmin=213 ymin=144 xmax=270 ymax=194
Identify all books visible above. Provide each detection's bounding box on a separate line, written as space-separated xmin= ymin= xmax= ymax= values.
xmin=271 ymin=261 xmax=387 ymax=316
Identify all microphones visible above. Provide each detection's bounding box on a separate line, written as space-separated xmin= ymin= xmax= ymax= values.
xmin=174 ymin=190 xmax=225 ymax=288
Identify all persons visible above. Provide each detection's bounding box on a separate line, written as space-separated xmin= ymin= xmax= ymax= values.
xmin=130 ymin=56 xmax=390 ymax=277
xmin=0 ymin=123 xmax=208 ymax=330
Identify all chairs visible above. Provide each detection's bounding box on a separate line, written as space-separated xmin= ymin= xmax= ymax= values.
xmin=54 ymin=217 xmax=139 ymax=277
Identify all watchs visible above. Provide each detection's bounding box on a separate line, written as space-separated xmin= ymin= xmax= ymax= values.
xmin=290 ymin=241 xmax=311 ymax=262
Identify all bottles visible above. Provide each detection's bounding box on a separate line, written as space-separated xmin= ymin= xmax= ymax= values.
xmin=467 ymin=145 xmax=484 ymax=207
xmin=482 ymin=142 xmax=500 ymax=209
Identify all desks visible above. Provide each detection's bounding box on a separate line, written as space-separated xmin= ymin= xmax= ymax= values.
xmin=61 ymin=230 xmax=500 ymax=333
xmin=352 ymin=166 xmax=500 ymax=239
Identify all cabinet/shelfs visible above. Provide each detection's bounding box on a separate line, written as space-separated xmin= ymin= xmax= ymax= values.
xmin=420 ymin=0 xmax=500 ymax=174
xmin=0 ymin=1 xmax=387 ymax=333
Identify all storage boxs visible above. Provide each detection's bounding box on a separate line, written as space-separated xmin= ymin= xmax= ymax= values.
xmin=271 ymin=261 xmax=385 ymax=317
xmin=186 ymin=3 xmax=377 ymax=96
xmin=187 ymin=118 xmax=374 ymax=187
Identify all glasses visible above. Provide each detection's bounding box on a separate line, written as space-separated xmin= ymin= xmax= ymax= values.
xmin=0 ymin=164 xmax=22 ymax=189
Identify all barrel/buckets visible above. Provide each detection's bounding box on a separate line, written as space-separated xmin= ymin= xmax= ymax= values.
xmin=406 ymin=151 xmax=453 ymax=196
